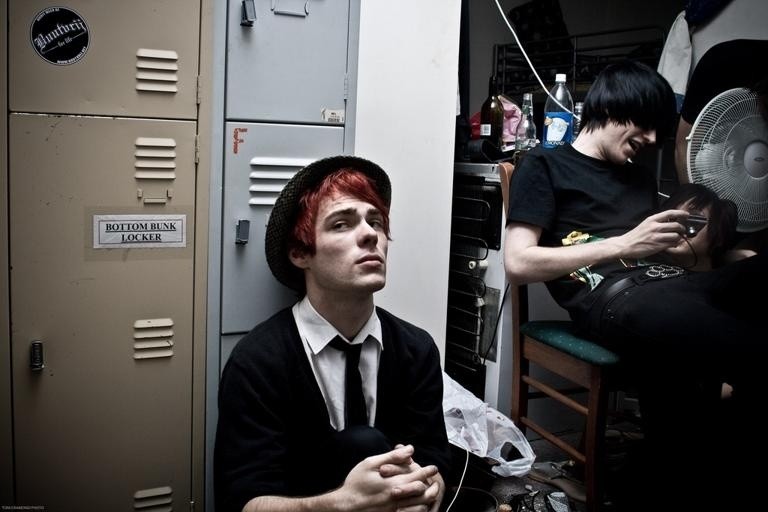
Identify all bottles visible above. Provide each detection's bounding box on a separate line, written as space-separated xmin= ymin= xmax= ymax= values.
xmin=515 ymin=92 xmax=538 ymax=151
xmin=479 ymin=75 xmax=504 ymax=150
xmin=542 ymin=72 xmax=575 ymax=149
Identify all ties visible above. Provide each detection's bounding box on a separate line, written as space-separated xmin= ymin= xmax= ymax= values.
xmin=328 ymin=335 xmax=367 ymax=426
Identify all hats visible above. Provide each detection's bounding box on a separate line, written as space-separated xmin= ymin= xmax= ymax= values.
xmin=265 ymin=156 xmax=391 ymax=291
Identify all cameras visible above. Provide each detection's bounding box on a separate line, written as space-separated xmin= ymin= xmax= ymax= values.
xmin=671 ymin=211 xmax=709 ymax=240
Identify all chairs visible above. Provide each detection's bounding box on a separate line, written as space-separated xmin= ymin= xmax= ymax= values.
xmin=498 ymin=162 xmax=623 ymax=511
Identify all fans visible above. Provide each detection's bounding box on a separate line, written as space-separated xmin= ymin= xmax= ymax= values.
xmin=684 ymin=87 xmax=768 ymax=235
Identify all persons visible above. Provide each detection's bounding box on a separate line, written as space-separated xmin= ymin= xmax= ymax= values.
xmin=660 ymin=185 xmax=758 ymax=300
xmin=504 ymin=58 xmax=763 ymax=508
xmin=211 ymin=157 xmax=452 ymax=511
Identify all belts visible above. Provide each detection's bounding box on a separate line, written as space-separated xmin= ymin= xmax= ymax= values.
xmin=593 ymin=265 xmax=684 ymax=329
